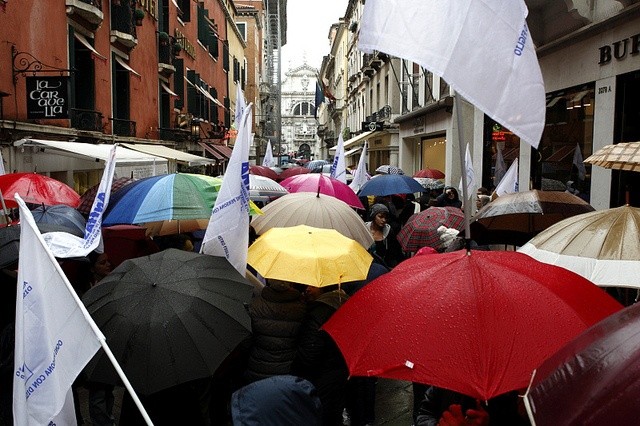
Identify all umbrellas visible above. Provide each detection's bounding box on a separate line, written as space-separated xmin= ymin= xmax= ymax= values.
xmin=81 ymin=247 xmax=254 ymax=399
xmin=413 ymin=166 xmax=445 ymax=179
xmin=472 ymin=188 xmax=597 ymax=240
xmin=0 ymin=165 xmax=82 ymax=208
xmin=348 ymin=169 xmax=371 ymax=181
xmin=249 ymin=138 xmax=331 ymax=182
xmin=358 ymin=167 xmax=429 ymax=203
xmin=412 ymin=177 xmax=444 ymax=190
xmin=75 ymin=170 xmax=145 ymax=216
xmin=251 ymin=186 xmax=373 ymax=253
xmin=375 ymin=165 xmax=404 ymax=175
xmin=247 ymin=224 xmax=374 ymax=290
xmin=317 ymin=238 xmax=615 ymax=417
xmin=395 ymin=205 xmax=465 ymax=253
xmin=102 ymin=170 xmax=266 ymax=234
xmin=581 ymin=141 xmax=639 ymax=172
xmin=515 ymin=203 xmax=640 ymax=292
xmin=517 ymin=301 xmax=639 ymax=426
xmin=215 ymin=172 xmax=289 ymax=197
xmin=0 ymin=223 xmax=84 ymax=270
xmin=278 ymin=164 xmax=366 ymax=210
xmin=29 ymin=201 xmax=86 ymax=238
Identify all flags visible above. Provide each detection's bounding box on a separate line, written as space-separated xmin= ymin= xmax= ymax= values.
xmin=315 ymin=81 xmax=325 ymax=107
xmin=12 ymin=194 xmax=107 ymax=426
xmin=40 ymin=141 xmax=117 ymax=260
xmin=231 ymin=80 xmax=249 ymax=131
xmin=356 ymin=0 xmax=546 ymax=150
xmin=330 ymin=133 xmax=347 ymax=184
xmin=347 ymin=140 xmax=367 ymax=194
xmin=495 ymin=157 xmax=518 ymax=196
xmin=319 ymin=75 xmax=336 ymax=100
xmin=197 ymin=102 xmax=254 ymax=278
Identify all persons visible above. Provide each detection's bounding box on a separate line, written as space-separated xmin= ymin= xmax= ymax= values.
xmin=415 ymin=189 xmax=434 ymax=203
xmin=436 ymin=225 xmax=460 ymax=252
xmin=475 ymin=195 xmax=490 ymax=209
xmin=414 ymin=246 xmax=437 ymax=257
xmin=85 ymin=382 xmax=115 ymax=426
xmin=416 ymin=387 xmax=531 ymax=426
xmin=87 ymin=250 xmax=112 ymax=287
xmin=345 ymin=264 xmax=388 ymax=423
xmin=475 ymin=187 xmax=490 ymax=197
xmin=390 ymin=197 xmax=411 ymax=227
xmin=364 ymin=204 xmax=404 ymax=270
xmin=300 ymin=285 xmax=350 ymax=426
xmin=247 ymin=278 xmax=309 ymax=379
xmin=433 ymin=185 xmax=462 ymax=208
xmin=404 ymin=193 xmax=417 ymax=206
xmin=231 ymin=375 xmax=323 ymax=425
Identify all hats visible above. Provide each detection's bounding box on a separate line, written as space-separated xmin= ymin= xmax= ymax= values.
xmin=369 ymin=203 xmax=392 ymax=220
xmin=437 ymin=225 xmax=460 ymax=249
